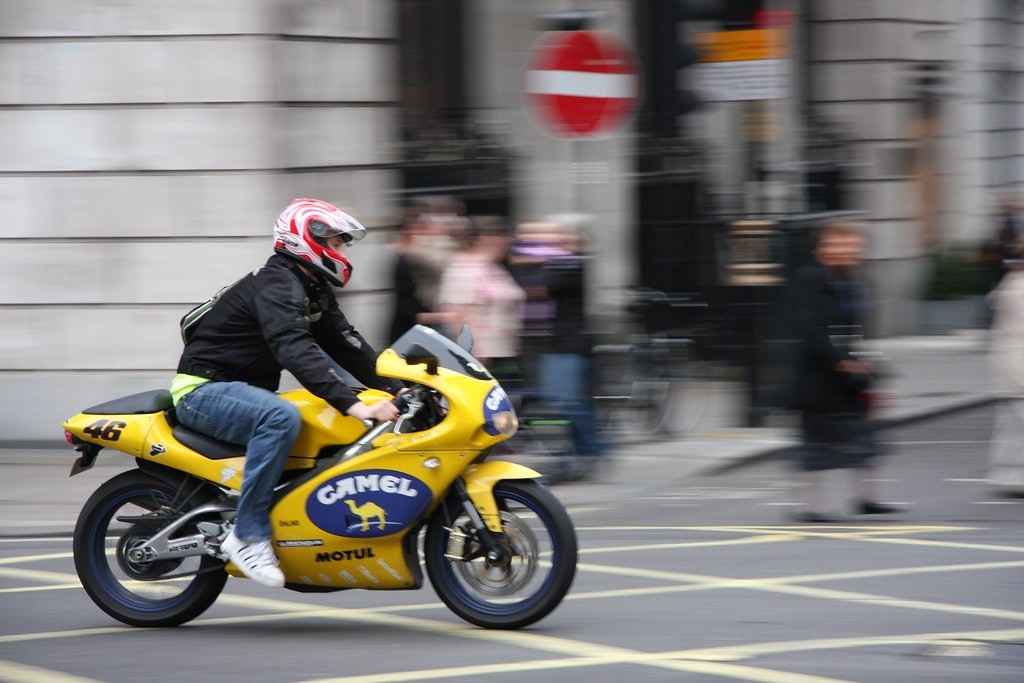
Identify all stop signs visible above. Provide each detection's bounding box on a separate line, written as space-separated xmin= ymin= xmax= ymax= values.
xmin=523 ymin=28 xmax=646 ymax=144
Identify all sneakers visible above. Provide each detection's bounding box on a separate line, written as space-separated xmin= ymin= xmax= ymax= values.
xmin=220 ymin=530 xmax=285 ymax=588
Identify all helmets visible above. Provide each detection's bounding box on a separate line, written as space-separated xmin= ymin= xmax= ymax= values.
xmin=272 ymin=198 xmax=354 ymax=287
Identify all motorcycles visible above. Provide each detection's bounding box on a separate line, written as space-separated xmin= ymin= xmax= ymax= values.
xmin=58 ymin=321 xmax=580 ymax=630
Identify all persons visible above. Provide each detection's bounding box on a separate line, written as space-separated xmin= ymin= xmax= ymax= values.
xmin=169 ymin=198 xmax=413 ymax=589
xmin=387 ymin=196 xmax=1024 ymax=519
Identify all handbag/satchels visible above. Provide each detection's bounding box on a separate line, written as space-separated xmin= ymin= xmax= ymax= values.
xmin=178 ymin=272 xmax=254 ymax=344
